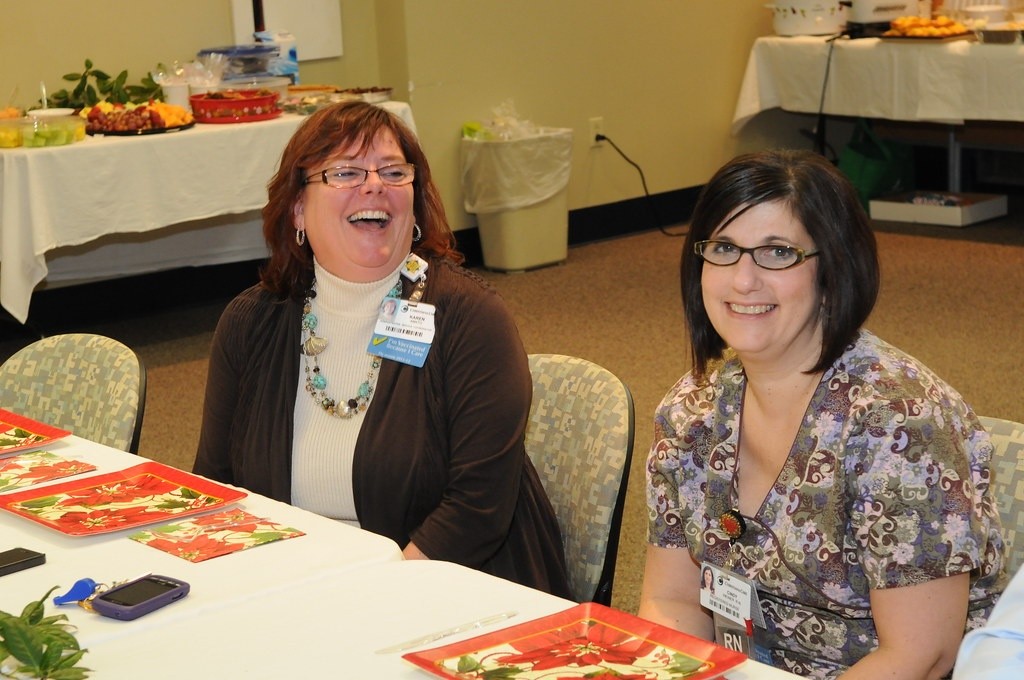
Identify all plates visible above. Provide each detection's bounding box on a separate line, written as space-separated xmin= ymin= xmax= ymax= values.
xmin=879 ymin=30 xmax=974 ymax=44
xmin=0 ymin=408 xmax=72 ymax=454
xmin=192 ymin=108 xmax=282 ymax=123
xmin=287 ymin=86 xmax=392 ymax=104
xmin=401 ymin=602 xmax=748 ymax=680
xmin=0 ymin=461 xmax=248 ymax=536
xmin=86 ymin=118 xmax=196 ymax=135
xmin=26 ymin=108 xmax=75 ymax=116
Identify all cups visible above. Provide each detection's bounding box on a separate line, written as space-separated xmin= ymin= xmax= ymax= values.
xmin=161 ymin=83 xmax=190 ymax=112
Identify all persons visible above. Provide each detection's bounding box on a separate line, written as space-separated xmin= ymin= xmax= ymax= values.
xmin=193 ymin=100 xmax=580 ymax=602
xmin=632 ymin=148 xmax=1010 ymax=680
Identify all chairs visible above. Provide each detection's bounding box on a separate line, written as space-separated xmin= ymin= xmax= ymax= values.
xmin=975 ymin=416 xmax=1023 ymax=579
xmin=0 ymin=332 xmax=146 ymax=455
xmin=525 ymin=353 xmax=638 ymax=608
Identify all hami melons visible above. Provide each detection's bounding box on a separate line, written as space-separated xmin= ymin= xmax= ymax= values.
xmin=152 ymin=103 xmax=194 ymax=127
xmin=884 ymin=14 xmax=971 ymax=36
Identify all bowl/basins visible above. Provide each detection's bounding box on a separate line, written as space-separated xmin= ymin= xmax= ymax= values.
xmin=195 ymin=42 xmax=292 ymax=103
xmin=0 ymin=115 xmax=86 ymax=147
xmin=958 ymin=5 xmax=1020 ymax=43
xmin=762 ymin=0 xmax=848 ymax=36
xmin=189 ymin=89 xmax=280 ymax=116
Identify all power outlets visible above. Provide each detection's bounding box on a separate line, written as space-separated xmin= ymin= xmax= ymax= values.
xmin=587 ymin=117 xmax=605 ymax=147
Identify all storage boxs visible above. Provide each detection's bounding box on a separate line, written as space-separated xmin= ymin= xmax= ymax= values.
xmin=870 ymin=190 xmax=1007 ymax=225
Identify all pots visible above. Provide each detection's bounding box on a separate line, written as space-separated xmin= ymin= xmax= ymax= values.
xmin=840 ymin=0 xmax=920 ymax=37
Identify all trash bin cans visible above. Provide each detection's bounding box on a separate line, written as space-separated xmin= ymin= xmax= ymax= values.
xmin=460 ymin=128 xmax=574 ymax=273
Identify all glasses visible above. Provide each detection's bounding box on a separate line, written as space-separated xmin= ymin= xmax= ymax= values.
xmin=303 ymin=164 xmax=418 ymax=190
xmin=694 ymin=239 xmax=825 ymax=271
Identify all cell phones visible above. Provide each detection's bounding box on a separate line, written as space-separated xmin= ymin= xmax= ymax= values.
xmin=90 ymin=575 xmax=190 ymax=622
xmin=0 ymin=548 xmax=46 ymax=577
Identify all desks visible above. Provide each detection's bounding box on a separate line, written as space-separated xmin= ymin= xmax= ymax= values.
xmin=0 ymin=99 xmax=419 ymax=324
xmin=730 ymin=29 xmax=1024 ymax=198
xmin=0 ymin=408 xmax=814 ymax=680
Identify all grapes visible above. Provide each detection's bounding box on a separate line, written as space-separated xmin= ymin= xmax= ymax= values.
xmin=83 ymin=105 xmax=165 ymax=131
